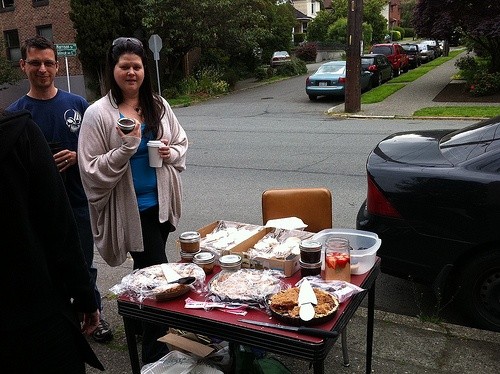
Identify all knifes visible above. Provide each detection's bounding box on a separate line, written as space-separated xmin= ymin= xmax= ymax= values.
xmin=237 ymin=317 xmax=338 ymax=338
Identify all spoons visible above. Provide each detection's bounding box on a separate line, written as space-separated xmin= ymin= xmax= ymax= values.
xmin=167 ymin=277 xmax=196 ymax=286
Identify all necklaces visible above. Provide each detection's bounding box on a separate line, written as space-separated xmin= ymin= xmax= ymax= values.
xmin=124 ymin=101 xmax=144 ymax=116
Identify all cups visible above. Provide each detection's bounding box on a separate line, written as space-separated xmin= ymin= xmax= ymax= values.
xmin=147 ymin=140 xmax=166 ymax=168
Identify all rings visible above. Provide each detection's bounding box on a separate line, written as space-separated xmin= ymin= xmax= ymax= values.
xmin=65 ymin=160 xmax=68 ymax=163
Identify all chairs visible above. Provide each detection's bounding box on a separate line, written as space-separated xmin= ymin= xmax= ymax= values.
xmin=261 ymin=189 xmax=350 ymax=367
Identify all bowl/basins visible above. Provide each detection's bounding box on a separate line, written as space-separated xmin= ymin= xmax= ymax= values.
xmin=141 ymin=350 xmax=197 ymax=374
xmin=310 ymin=227 xmax=382 ymax=276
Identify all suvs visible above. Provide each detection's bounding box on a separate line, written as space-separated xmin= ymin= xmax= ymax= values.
xmin=449 ymin=30 xmax=462 ymax=45
xmin=369 ymin=42 xmax=410 ymax=76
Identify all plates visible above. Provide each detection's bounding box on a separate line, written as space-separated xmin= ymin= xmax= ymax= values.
xmin=269 ymin=287 xmax=338 ymax=318
xmin=127 ymin=263 xmax=206 ymax=295
xmin=208 ymin=270 xmax=281 ymax=301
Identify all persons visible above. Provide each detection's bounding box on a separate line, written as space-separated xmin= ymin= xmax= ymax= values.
xmin=0 ymin=108 xmax=105 ymax=374
xmin=76 ymin=38 xmax=189 ymax=272
xmin=6 ymin=37 xmax=114 ymax=345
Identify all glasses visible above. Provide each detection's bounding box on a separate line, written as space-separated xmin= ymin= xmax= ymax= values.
xmin=111 ymin=37 xmax=143 ymax=53
xmin=23 ymin=58 xmax=58 ymax=69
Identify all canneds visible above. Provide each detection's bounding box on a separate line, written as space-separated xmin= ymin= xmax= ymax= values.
xmin=116 ymin=117 xmax=135 ymax=134
xmin=325 ymin=237 xmax=351 ymax=284
xmin=178 ymin=231 xmax=242 ymax=274
xmin=299 ymin=240 xmax=322 ymax=277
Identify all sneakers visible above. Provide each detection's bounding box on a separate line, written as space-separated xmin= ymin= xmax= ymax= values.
xmin=90 ymin=317 xmax=114 ymax=342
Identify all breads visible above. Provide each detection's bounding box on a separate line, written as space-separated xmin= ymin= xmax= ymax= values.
xmin=254 ymin=236 xmax=301 ymax=258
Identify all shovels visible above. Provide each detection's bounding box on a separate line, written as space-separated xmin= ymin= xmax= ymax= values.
xmin=161 ymin=263 xmax=196 ymax=285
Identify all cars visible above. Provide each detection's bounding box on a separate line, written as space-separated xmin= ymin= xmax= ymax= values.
xmin=270 ymin=51 xmax=291 ymax=67
xmin=353 ymin=112 xmax=500 ymax=331
xmin=361 ymin=53 xmax=395 ymax=86
xmin=400 ymin=40 xmax=449 ymax=69
xmin=305 ymin=60 xmax=374 ymax=101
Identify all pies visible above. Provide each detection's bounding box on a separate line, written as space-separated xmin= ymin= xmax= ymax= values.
xmin=270 ymin=287 xmax=338 ymax=318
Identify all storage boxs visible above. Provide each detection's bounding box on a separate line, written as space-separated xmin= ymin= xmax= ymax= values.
xmin=310 ymin=229 xmax=382 ymax=275
xmin=176 ymin=220 xmax=265 ymax=257
xmin=157 ymin=329 xmax=215 ymax=358
xmin=232 ymin=227 xmax=316 ymax=277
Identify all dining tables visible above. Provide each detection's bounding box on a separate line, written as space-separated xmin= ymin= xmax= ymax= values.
xmin=117 ymin=255 xmax=381 ymax=374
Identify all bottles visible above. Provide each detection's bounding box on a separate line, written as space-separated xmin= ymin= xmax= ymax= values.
xmin=323 ymin=236 xmax=350 ymax=284
